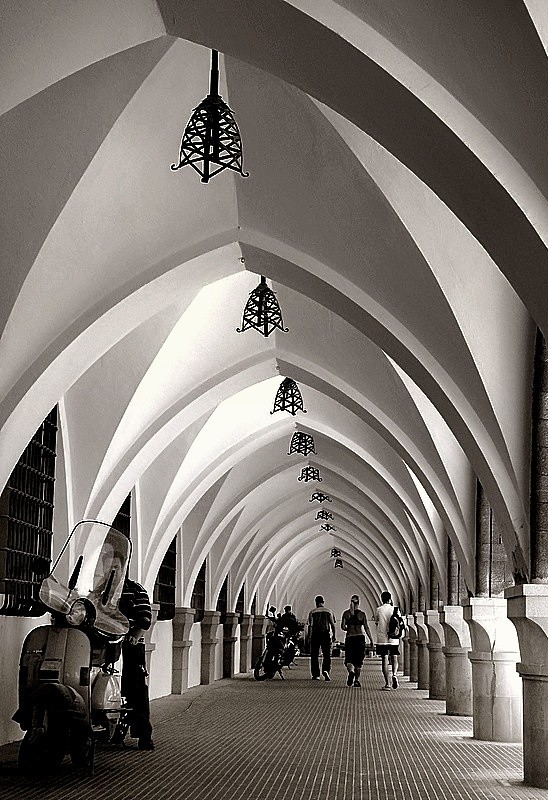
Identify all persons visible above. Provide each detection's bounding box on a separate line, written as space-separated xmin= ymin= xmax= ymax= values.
xmin=85 ymin=550 xmax=157 ymax=750
xmin=279 ymin=605 xmax=298 ymax=633
xmin=372 ymin=591 xmax=407 ymax=691
xmin=305 ymin=595 xmax=338 ymax=682
xmin=341 ymin=594 xmax=375 ymax=687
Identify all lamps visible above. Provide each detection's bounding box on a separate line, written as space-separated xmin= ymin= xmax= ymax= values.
xmin=169 ymin=49 xmax=249 ymax=185
xmin=286 ymin=428 xmax=344 ymax=569
xmin=270 ymin=377 xmax=307 ymax=416
xmin=236 ymin=274 xmax=289 ymax=337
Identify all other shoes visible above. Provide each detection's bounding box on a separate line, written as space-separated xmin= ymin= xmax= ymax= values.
xmin=392 ymin=675 xmax=398 ymax=689
xmin=323 ymin=670 xmax=331 ymax=681
xmin=138 ymin=739 xmax=155 ymax=751
xmin=382 ymin=686 xmax=391 ymax=691
xmin=311 ymin=674 xmax=319 ymax=680
xmin=347 ymin=671 xmax=355 ymax=686
xmin=354 ymin=681 xmax=362 ymax=687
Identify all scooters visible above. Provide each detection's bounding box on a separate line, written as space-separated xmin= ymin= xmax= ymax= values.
xmin=11 ymin=518 xmax=133 ymax=781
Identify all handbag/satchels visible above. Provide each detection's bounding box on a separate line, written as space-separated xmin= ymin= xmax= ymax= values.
xmin=387 ymin=607 xmax=405 ymax=641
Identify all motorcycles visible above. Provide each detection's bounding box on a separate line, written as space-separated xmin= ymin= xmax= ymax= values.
xmin=254 ymin=606 xmax=302 ymax=680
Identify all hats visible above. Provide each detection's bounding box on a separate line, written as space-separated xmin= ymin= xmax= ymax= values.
xmin=284 ymin=606 xmax=291 ymax=611
